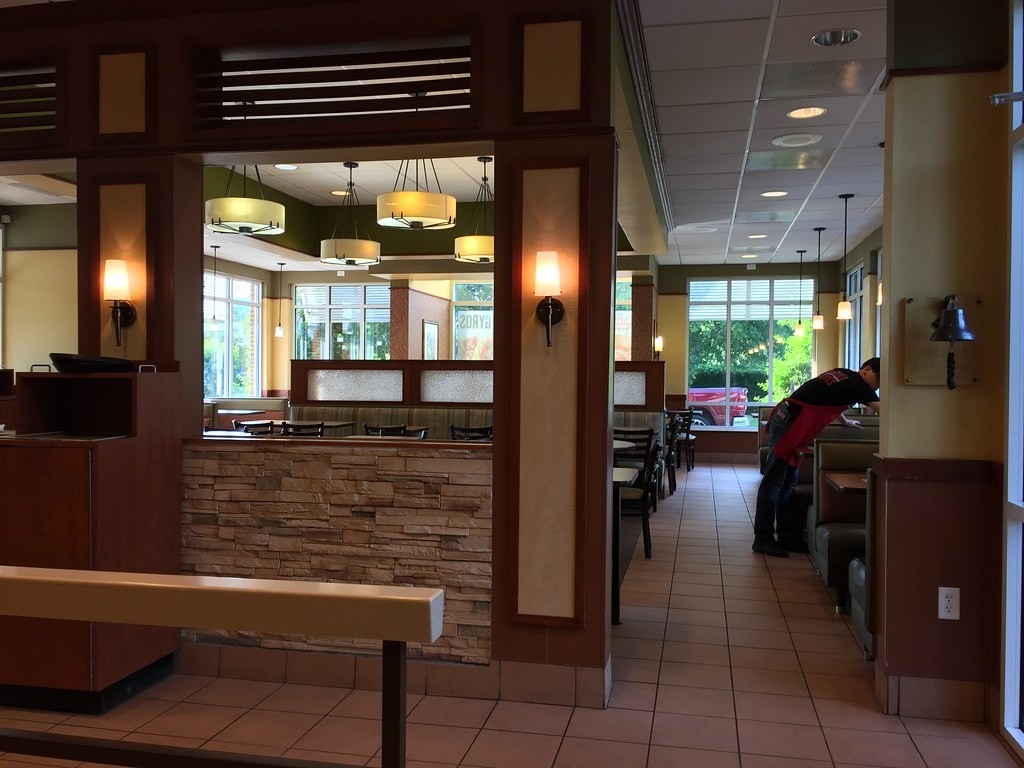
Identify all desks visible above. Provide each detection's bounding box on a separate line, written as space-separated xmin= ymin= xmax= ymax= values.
xmin=203 ymin=395 xmax=869 ymax=613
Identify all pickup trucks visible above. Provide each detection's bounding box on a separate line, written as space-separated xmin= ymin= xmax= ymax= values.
xmin=688 ymin=387 xmax=748 ymax=426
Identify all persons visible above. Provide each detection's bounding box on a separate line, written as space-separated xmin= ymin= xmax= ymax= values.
xmin=751 ymin=356 xmax=881 ymax=559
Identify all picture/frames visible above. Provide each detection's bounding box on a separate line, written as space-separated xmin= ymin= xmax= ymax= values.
xmin=422 ymin=318 xmax=439 ymax=359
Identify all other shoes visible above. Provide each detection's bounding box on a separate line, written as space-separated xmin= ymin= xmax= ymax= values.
xmin=752 ymin=535 xmax=790 ymax=558
xmin=777 ymin=537 xmax=812 ymax=555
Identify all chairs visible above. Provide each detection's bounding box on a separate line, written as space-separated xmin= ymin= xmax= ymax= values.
xmin=379 ymin=430 xmax=428 ymax=439
xmin=448 ymin=424 xmax=492 ymax=440
xmin=622 ymin=439 xmax=659 ymax=560
xmin=665 ymin=410 xmax=693 ymax=472
xmin=281 ymin=422 xmax=325 ymax=437
xmin=362 ymin=423 xmax=407 ymax=436
xmin=231 ymin=419 xmax=274 ymax=435
xmin=662 ymin=413 xmax=681 ymax=494
xmin=613 ymin=427 xmax=654 ymax=518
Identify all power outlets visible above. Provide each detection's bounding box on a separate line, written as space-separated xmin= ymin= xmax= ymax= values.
xmin=938 ymin=587 xmax=961 ymax=620
xmin=970 ymin=368 xmax=992 ymax=395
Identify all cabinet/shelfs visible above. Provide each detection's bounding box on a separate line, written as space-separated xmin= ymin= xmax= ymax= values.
xmin=0 ymin=372 xmax=184 ymax=715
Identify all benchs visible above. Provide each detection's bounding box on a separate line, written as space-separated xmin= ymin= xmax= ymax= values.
xmin=758 ymin=407 xmax=879 ymax=589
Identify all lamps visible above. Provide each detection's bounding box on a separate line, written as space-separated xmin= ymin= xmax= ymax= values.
xmin=273 ymin=262 xmax=286 ymax=337
xmin=209 ymin=245 xmax=221 ymax=332
xmin=876 ymin=142 xmax=885 ymax=306
xmin=103 ymin=259 xmax=137 ymax=347
xmin=794 ymin=250 xmax=807 ymax=336
xmin=835 ymin=194 xmax=855 ymax=320
xmin=454 ymin=156 xmax=495 ymax=264
xmin=655 ymin=336 xmax=663 ymax=361
xmin=534 ymin=250 xmax=563 ymax=346
xmin=320 ymin=162 xmax=380 ymax=266
xmin=337 ymin=333 xmax=344 ymax=342
xmin=204 ymin=101 xmax=286 ymax=235
xmin=812 ymin=227 xmax=826 ymax=329
xmin=376 ymin=91 xmax=458 ymax=231
xmin=777 ymin=333 xmax=783 ymax=344
xmin=759 ymin=340 xmax=766 ymax=349
xmin=749 ymin=346 xmax=759 ymax=354
